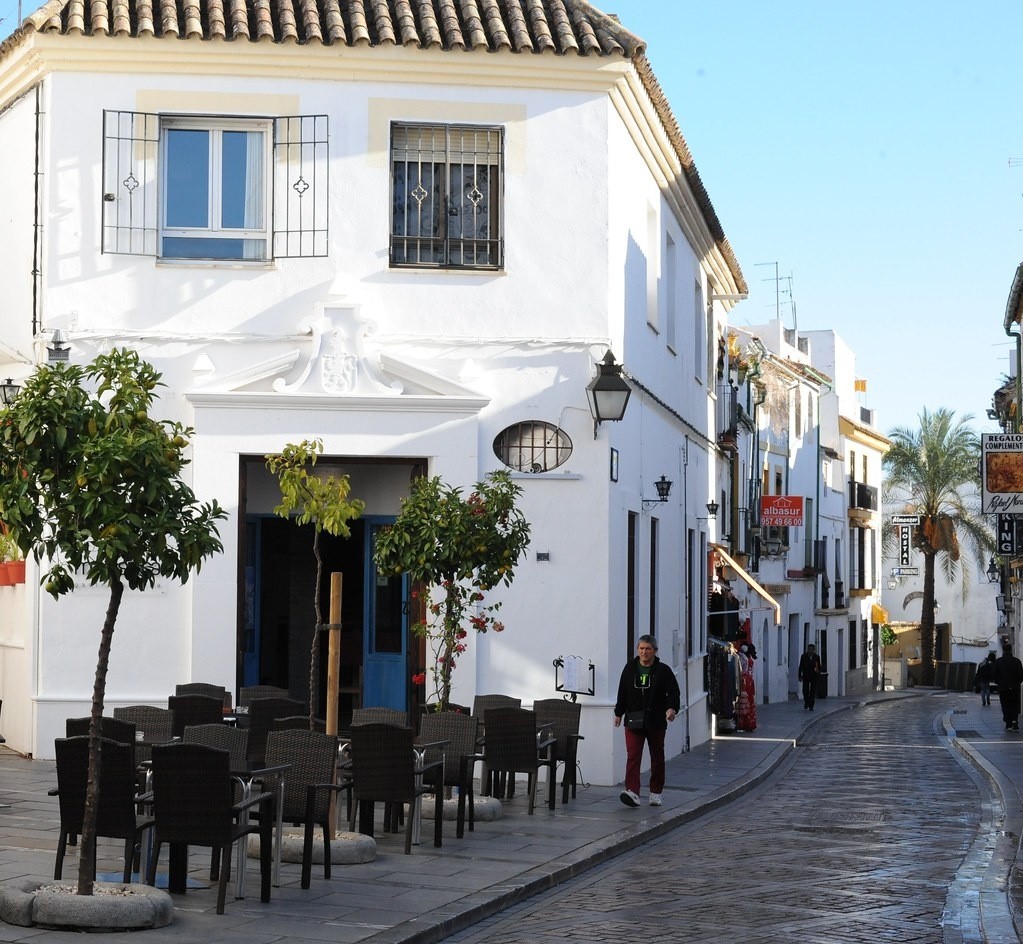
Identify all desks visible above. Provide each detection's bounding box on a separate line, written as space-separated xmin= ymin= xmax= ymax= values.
xmin=479 ymin=720 xmax=558 ymax=809
xmin=134 ymin=733 xmax=181 ymax=747
xmin=334 ymin=734 xmax=451 ymax=845
xmin=139 ymin=759 xmax=294 ymax=900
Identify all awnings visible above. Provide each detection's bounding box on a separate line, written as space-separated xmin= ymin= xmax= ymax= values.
xmin=707 ymin=541 xmax=781 ymax=627
xmin=871 ymin=603 xmax=888 ymax=623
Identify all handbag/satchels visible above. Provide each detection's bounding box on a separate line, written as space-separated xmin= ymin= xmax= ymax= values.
xmin=626 ymin=711 xmax=645 ymax=730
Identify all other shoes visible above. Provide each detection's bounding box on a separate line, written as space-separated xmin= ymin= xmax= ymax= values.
xmin=982 ymin=700 xmax=986 ymax=705
xmin=809 ymin=709 xmax=814 ymax=711
xmin=987 ymin=697 xmax=990 ymax=705
xmin=1012 ymin=725 xmax=1020 ymax=731
xmin=1006 ymin=723 xmax=1012 ymax=731
xmin=804 ymin=703 xmax=808 ymax=709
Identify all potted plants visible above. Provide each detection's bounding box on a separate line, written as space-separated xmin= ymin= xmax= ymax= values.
xmin=0 ymin=526 xmax=26 ymax=587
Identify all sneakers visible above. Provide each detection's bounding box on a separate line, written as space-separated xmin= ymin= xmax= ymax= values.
xmin=648 ymin=792 xmax=664 ymax=806
xmin=619 ymin=788 xmax=640 ymax=807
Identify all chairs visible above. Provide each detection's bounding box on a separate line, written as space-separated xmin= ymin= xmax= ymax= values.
xmin=148 ymin=742 xmax=273 ymax=914
xmin=68 ymin=683 xmax=583 ymax=855
xmin=253 ymin=728 xmax=342 ymax=889
xmin=48 ymin=735 xmax=156 ymax=885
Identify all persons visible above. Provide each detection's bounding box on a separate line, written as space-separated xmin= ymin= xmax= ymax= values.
xmin=614 ymin=635 xmax=680 ymax=807
xmin=992 ymin=644 xmax=1023 ymax=731
xmin=798 ymin=644 xmax=820 ymax=711
xmin=978 ymin=658 xmax=992 ymax=705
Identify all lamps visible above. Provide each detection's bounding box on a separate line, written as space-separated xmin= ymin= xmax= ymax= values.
xmin=996 ymin=592 xmax=1008 ymax=616
xmin=933 ymin=598 xmax=941 ymax=614
xmin=882 ymin=571 xmax=897 ymax=590
xmin=0 ymin=377 xmax=20 ymax=408
xmin=584 ymin=349 xmax=633 ymax=438
xmin=697 ymin=500 xmax=719 ymax=519
xmin=760 ymin=524 xmax=781 ymax=555
xmin=642 ymin=474 xmax=673 ymax=502
xmin=986 ymin=557 xmax=1005 ymax=584
